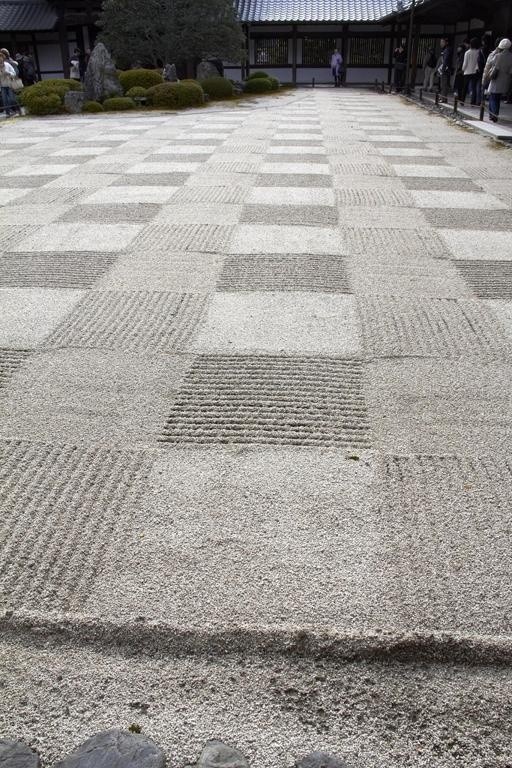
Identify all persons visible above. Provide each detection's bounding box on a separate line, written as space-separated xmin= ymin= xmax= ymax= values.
xmin=422 ymin=36 xmax=512 ymax=123
xmin=391 ymin=43 xmax=406 ymax=92
xmin=330 ymin=47 xmax=343 ymax=87
xmin=69 ymin=48 xmax=92 ymax=81
xmin=0 ymin=48 xmax=37 ymax=118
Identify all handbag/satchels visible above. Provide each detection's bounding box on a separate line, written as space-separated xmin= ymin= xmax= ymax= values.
xmin=338 ymin=66 xmax=345 ymax=74
xmin=477 ymin=54 xmax=484 ymax=73
xmin=11 ymin=79 xmax=24 ymax=90
xmin=488 ymin=67 xmax=500 ymax=82
xmin=397 ymin=63 xmax=405 ymax=71
xmin=333 ymin=67 xmax=336 ymax=76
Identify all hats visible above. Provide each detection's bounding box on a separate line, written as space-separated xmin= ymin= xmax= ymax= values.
xmin=498 ymin=38 xmax=511 ymax=49
xmin=15 ymin=53 xmax=23 ymax=60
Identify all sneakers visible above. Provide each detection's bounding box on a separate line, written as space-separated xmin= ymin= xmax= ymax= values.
xmin=489 ymin=114 xmax=497 ymax=122
xmin=438 ymin=97 xmax=447 ymax=103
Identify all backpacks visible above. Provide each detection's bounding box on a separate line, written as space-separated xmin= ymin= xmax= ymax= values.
xmin=427 ymin=53 xmax=437 ymax=68
